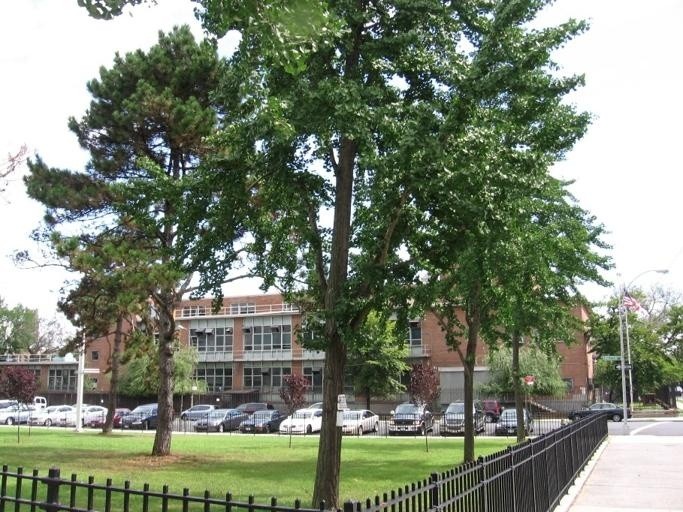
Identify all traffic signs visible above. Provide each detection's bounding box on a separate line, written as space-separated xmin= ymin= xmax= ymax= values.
xmin=613 ymin=363 xmax=633 ymax=370
xmin=602 ymin=355 xmax=622 ymax=362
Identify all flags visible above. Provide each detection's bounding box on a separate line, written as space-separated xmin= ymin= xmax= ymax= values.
xmin=622 ymin=287 xmax=639 ymax=313
xmin=633 ymin=305 xmax=649 ymax=320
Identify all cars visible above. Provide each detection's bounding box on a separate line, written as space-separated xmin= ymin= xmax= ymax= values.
xmin=495 ymin=407 xmax=536 ymax=436
xmin=566 ymin=401 xmax=632 ymax=423
xmin=675 ymin=385 xmax=682 ymax=396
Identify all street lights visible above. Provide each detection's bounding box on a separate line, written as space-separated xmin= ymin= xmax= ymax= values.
xmin=611 ymin=267 xmax=669 ymax=433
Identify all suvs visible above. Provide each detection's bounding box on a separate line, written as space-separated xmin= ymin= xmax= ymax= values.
xmin=483 ymin=398 xmax=504 ymax=423
xmin=386 ymin=401 xmax=435 ymax=435
xmin=438 ymin=398 xmax=487 ymax=436
